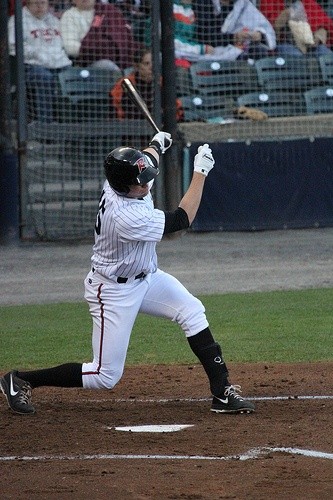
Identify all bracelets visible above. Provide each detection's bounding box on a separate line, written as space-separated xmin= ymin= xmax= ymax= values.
xmin=149 ymin=145 xmax=159 ymax=154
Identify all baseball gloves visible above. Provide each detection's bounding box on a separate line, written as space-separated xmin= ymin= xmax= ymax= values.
xmin=233 ymin=106 xmax=267 ymax=121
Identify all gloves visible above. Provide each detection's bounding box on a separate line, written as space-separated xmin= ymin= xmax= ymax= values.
xmin=193 ymin=144 xmax=215 ymax=176
xmin=150 ymin=131 xmax=172 ymax=154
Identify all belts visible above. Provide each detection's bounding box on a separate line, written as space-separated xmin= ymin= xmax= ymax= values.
xmin=92 ymin=267 xmax=144 ymax=283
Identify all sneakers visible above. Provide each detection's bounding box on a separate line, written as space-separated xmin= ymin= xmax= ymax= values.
xmin=0 ymin=369 xmax=35 ymax=413
xmin=211 ymin=384 xmax=254 ymax=413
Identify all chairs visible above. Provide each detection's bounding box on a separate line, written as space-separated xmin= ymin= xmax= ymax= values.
xmin=57 ymin=55 xmax=333 ymax=180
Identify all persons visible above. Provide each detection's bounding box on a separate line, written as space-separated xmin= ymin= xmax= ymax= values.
xmin=260 ymin=0 xmax=333 ymax=48
xmin=109 ymin=45 xmax=184 ymax=122
xmin=0 ymin=132 xmax=255 ymax=414
xmin=8 ymin=0 xmax=277 ymax=70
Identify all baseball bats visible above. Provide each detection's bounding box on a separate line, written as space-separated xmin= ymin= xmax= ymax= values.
xmin=120 ymin=78 xmax=170 ymax=149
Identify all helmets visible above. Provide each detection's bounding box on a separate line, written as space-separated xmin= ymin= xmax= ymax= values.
xmin=104 ymin=146 xmax=160 ymax=196
